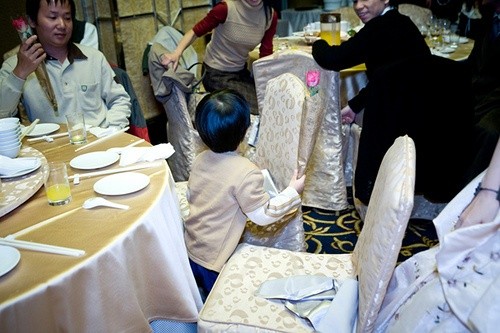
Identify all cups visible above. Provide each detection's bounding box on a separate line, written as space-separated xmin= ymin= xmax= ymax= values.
xmin=65 ymin=113 xmax=88 ymax=145
xmin=272 ymin=27 xmax=318 ymax=52
xmin=414 ymin=18 xmax=460 ymax=48
xmin=41 ymin=161 xmax=72 ymax=206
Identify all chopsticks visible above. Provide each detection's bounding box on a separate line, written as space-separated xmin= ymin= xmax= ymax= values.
xmin=26 ymin=133 xmax=69 ymax=141
xmin=0 ymin=237 xmax=86 ymax=257
xmin=68 ymin=163 xmax=153 ymax=179
xmin=75 ymin=127 xmax=130 ymax=153
xmin=20 ymin=118 xmax=40 ymax=140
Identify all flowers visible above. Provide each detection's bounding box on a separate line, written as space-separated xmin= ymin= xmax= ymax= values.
xmin=13 ymin=14 xmax=58 ymax=112
xmin=298 ymin=69 xmax=325 ymax=179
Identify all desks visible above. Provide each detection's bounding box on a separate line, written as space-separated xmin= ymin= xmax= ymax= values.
xmin=249 ymin=33 xmax=474 ymax=105
xmin=0 ymin=124 xmax=204 ymax=333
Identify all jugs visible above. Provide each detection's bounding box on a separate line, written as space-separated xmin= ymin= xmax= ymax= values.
xmin=320 ymin=13 xmax=341 ymax=46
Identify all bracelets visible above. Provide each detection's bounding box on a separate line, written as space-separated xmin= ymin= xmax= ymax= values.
xmin=474 ymin=183 xmax=500 ymax=201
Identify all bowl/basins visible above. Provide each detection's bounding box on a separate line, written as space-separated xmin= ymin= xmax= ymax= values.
xmin=0 ymin=117 xmax=23 ymax=159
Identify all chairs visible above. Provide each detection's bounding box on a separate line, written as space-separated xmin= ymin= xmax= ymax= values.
xmin=254 ymin=50 xmax=347 ymax=211
xmin=163 ymin=83 xmax=197 ymax=181
xmin=175 ymin=74 xmax=325 ymax=252
xmin=197 ymin=135 xmax=417 ymax=333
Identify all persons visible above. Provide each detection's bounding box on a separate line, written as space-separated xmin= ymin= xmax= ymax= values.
xmin=161 ymin=0 xmax=278 ymax=115
xmin=0 ymin=0 xmax=132 ymax=131
xmin=455 ymin=137 xmax=500 ymax=228
xmin=182 ymin=88 xmax=306 ymax=293
xmin=304 ymin=0 xmax=435 ymax=207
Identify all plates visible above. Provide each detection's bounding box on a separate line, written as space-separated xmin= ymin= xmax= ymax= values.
xmin=0 ymin=157 xmax=42 ymax=179
xmin=430 ymin=47 xmax=456 ymax=53
xmin=444 ymin=38 xmax=469 ymax=43
xmin=69 ymin=151 xmax=120 ymax=170
xmin=21 ymin=123 xmax=61 ymax=137
xmin=93 ymin=172 xmax=150 ymax=195
xmin=0 ymin=245 xmax=20 ymax=277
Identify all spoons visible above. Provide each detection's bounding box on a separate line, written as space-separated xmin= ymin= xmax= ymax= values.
xmin=82 ymin=197 xmax=129 ymax=210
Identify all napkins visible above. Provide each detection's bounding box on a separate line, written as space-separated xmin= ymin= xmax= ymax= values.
xmin=120 ymin=140 xmax=175 ymax=166
